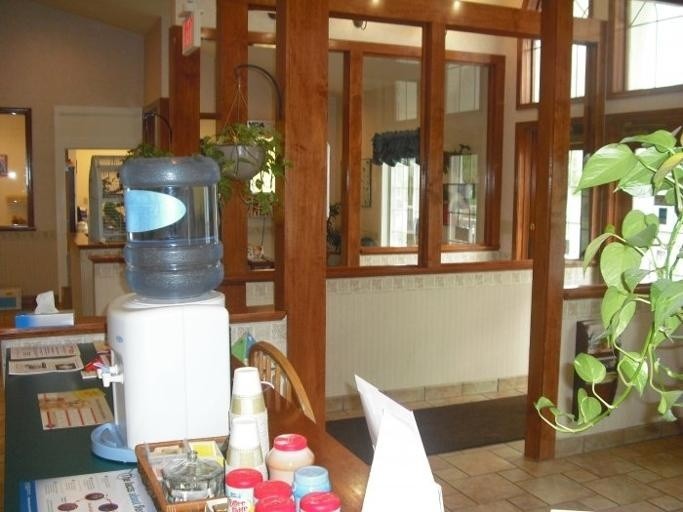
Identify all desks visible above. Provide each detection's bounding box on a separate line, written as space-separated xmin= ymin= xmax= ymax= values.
xmin=3 ymin=343 xmax=372 ymax=511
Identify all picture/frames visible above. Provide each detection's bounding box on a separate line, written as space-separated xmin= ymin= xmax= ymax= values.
xmin=0 ymin=106 xmax=36 ymax=231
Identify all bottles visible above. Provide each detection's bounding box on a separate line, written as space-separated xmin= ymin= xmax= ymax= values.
xmin=226 ymin=432 xmax=342 ymax=511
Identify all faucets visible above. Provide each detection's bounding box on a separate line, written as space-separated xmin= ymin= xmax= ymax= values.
xmin=84 ymin=355 xmax=119 ymax=378
xmin=95 ymin=362 xmax=124 ymax=389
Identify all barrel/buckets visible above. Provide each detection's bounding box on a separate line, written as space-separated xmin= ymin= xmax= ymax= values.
xmin=119 ymin=156 xmax=225 ymax=304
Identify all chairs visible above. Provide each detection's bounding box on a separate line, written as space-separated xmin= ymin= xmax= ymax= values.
xmin=247 ymin=340 xmax=315 ymax=425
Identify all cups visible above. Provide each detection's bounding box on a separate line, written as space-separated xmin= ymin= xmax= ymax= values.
xmin=225 ymin=365 xmax=271 ymax=493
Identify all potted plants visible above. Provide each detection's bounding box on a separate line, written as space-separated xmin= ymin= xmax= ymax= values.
xmin=199 ymin=120 xmax=292 ymax=215
